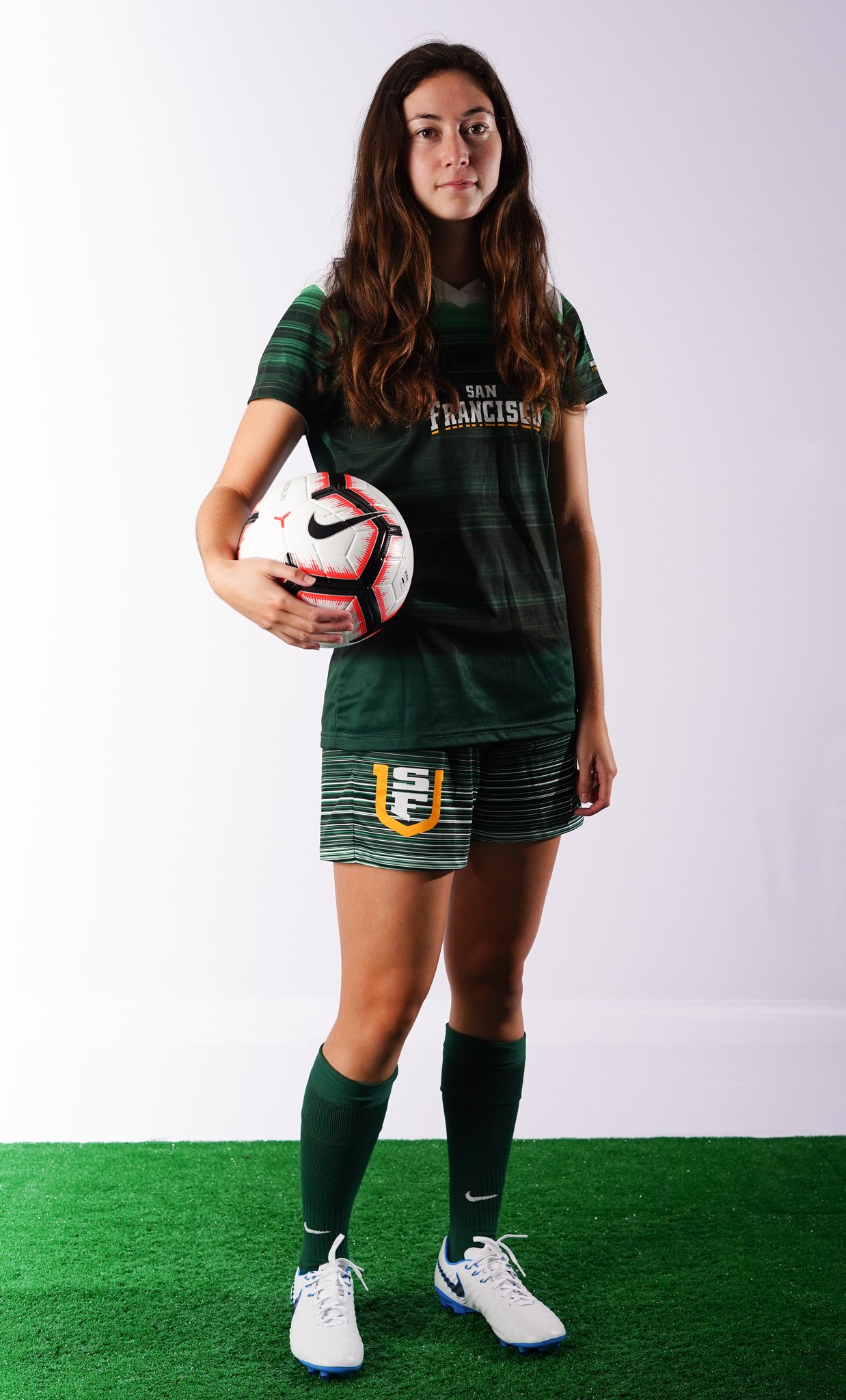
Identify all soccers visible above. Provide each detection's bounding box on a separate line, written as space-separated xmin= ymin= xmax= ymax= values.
xmin=234 ymin=471 xmax=414 ymax=649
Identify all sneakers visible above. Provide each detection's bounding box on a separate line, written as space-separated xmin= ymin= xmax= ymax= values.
xmin=433 ymin=1233 xmax=568 ymax=1354
xmin=288 ymin=1233 xmax=371 ymax=1379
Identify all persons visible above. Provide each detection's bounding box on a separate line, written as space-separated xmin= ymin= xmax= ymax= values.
xmin=191 ymin=37 xmax=618 ymax=1371
